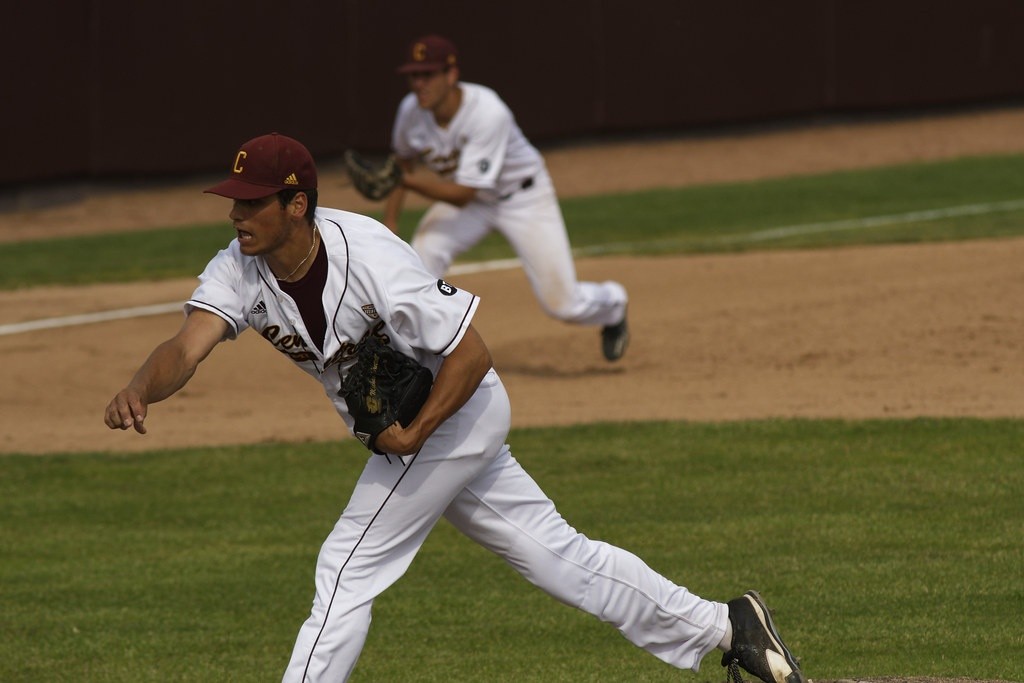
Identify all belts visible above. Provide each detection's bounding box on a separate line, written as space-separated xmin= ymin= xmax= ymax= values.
xmin=502 ymin=178 xmax=533 ymax=200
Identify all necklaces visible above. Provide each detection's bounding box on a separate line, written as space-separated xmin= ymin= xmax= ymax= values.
xmin=275 ymin=224 xmax=316 ymax=281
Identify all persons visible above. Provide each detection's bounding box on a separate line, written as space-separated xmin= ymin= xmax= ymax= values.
xmin=101 ymin=133 xmax=808 ymax=683
xmin=343 ymin=35 xmax=630 ymax=362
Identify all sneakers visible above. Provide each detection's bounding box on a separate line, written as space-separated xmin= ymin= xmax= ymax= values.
xmin=721 ymin=590 xmax=804 ymax=683
xmin=602 ymin=302 xmax=629 ymax=360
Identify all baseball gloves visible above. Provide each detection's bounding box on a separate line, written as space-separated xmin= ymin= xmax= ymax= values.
xmin=341 ymin=149 xmax=401 ymax=201
xmin=335 ymin=335 xmax=435 ymax=467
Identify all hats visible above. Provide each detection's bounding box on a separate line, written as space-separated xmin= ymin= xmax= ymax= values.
xmin=396 ymin=36 xmax=456 ymax=73
xmin=203 ymin=132 xmax=317 ymax=201
